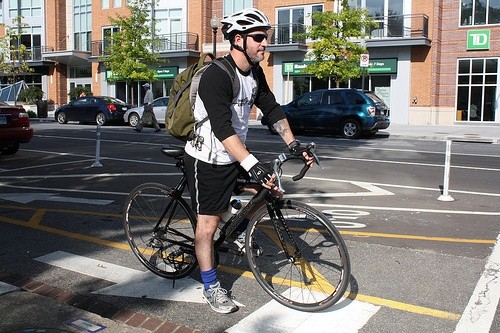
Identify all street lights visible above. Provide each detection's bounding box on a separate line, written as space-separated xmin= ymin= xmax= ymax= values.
xmin=210 ymin=15 xmax=221 ymax=58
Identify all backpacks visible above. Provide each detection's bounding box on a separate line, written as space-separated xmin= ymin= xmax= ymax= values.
xmin=166 ymin=54 xmax=239 ymax=142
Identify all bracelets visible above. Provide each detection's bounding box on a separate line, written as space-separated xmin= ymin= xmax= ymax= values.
xmin=240 ymin=154 xmax=259 ymax=172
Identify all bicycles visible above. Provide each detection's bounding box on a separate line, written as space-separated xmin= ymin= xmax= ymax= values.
xmin=124 ymin=140 xmax=352 ymax=312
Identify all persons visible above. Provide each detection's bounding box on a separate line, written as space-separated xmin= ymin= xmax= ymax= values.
xmin=80 ymin=89 xmax=86 ymax=97
xmin=132 ymin=83 xmax=161 ymax=133
xmin=183 ymin=8 xmax=314 ymax=314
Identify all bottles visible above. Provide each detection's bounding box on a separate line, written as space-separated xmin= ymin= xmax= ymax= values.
xmin=217 ymin=198 xmax=242 ymax=230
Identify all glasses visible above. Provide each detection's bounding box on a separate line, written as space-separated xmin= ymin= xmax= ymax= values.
xmin=247 ymin=33 xmax=269 ymax=42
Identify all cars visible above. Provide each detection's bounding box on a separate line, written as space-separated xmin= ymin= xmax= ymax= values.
xmin=0 ymin=99 xmax=34 ymax=154
xmin=123 ymin=96 xmax=170 ymax=127
xmin=54 ymin=95 xmax=137 ymax=126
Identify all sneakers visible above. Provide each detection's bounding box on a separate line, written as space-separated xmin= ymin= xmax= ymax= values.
xmin=216 ymin=220 xmax=263 ymax=255
xmin=202 ymin=282 xmax=238 ymax=314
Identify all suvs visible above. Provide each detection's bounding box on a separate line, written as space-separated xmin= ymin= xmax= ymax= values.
xmin=261 ymin=87 xmax=391 ymax=140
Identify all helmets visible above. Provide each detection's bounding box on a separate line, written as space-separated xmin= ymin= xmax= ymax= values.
xmin=221 ymin=9 xmax=272 ymax=40
